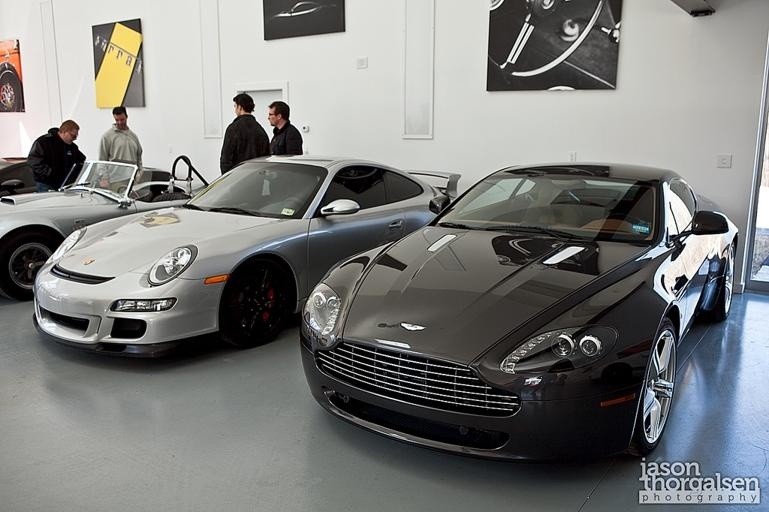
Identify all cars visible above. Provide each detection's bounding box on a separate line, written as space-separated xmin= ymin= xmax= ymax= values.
xmin=0 ymin=48 xmax=25 ymax=112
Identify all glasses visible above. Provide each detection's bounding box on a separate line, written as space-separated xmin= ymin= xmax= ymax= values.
xmin=268 ymin=113 xmax=278 ymax=116
xmin=68 ymin=131 xmax=77 ymax=138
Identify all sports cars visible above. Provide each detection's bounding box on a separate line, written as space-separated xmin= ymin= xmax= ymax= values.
xmin=266 ymin=0 xmax=338 ymax=30
xmin=275 ymin=153 xmax=740 ymax=464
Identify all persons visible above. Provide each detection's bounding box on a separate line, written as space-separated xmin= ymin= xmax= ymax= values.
xmin=267 ymin=100 xmax=303 ymax=193
xmin=98 ymin=105 xmax=144 ymax=194
xmin=27 ymin=119 xmax=86 ymax=192
xmin=219 ymin=93 xmax=270 ymax=197
